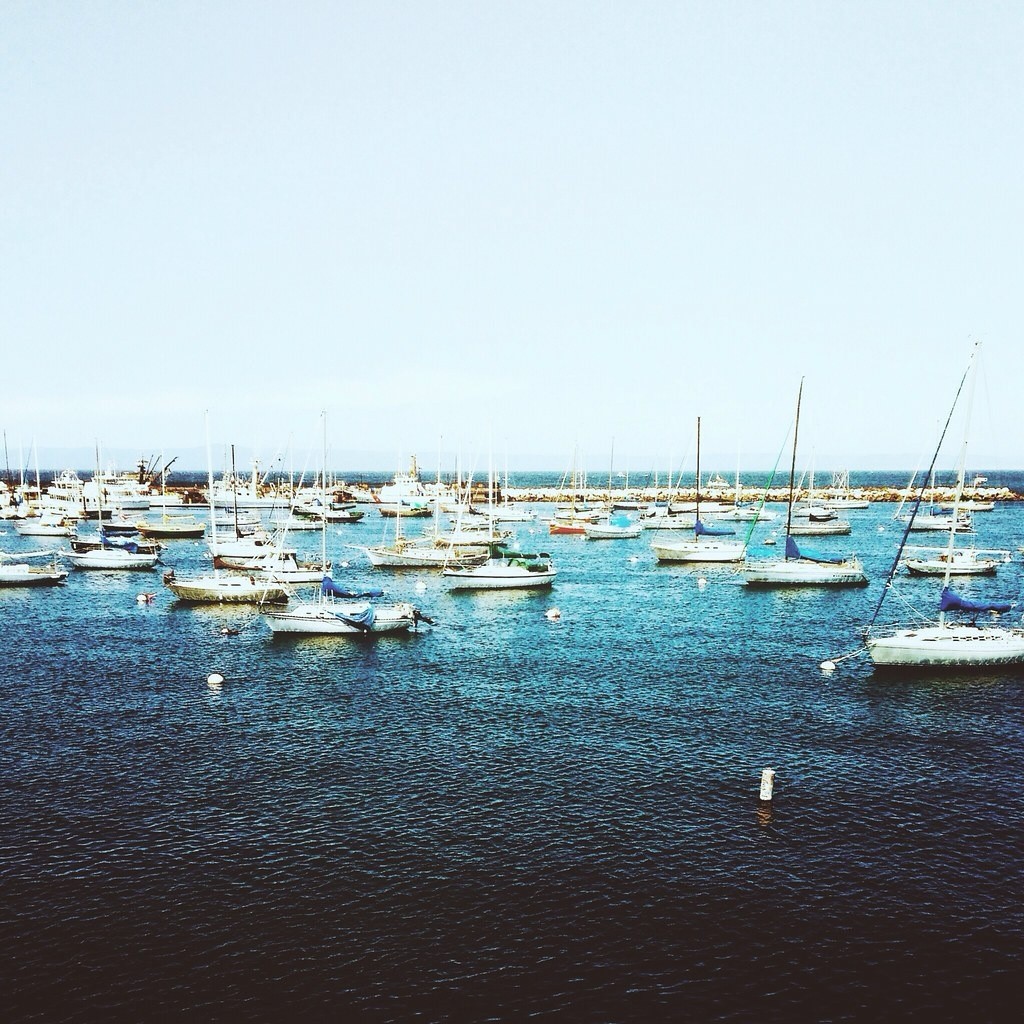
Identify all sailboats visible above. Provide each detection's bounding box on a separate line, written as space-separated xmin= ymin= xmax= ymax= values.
xmin=648 ymin=415 xmax=748 ymax=559
xmin=897 ymin=450 xmax=973 ymax=532
xmin=159 ymin=472 xmax=291 ymax=604
xmin=790 ymin=449 xmax=837 ymax=519
xmin=69 ymin=531 xmax=160 ymax=554
xmin=641 ymin=459 xmax=696 ymax=530
xmin=58 ymin=537 xmax=158 ymax=568
xmin=91 ymin=445 xmax=156 ymax=482
xmin=42 ymin=494 xmax=113 ymax=519
xmin=905 ymin=518 xmax=998 ymax=578
xmin=0 ymin=555 xmax=68 ymax=583
xmin=714 ymin=471 xmax=781 ymax=522
xmin=668 ymin=462 xmax=742 ymax=513
xmin=204 ymin=458 xmax=363 ymax=511
xmin=549 ymin=434 xmax=583 ymax=535
xmin=230 ymin=558 xmax=338 ymax=580
xmin=442 ymin=435 xmax=558 ymax=589
xmin=450 ymin=476 xmax=501 ymax=529
xmin=89 ymin=467 xmax=115 ymax=478
xmin=744 ymin=370 xmax=870 ymax=587
xmin=212 ymin=532 xmax=298 ymax=569
xmin=4 ymin=484 xmax=44 ymax=517
xmin=823 ymin=462 xmax=870 ymax=509
xmin=0 ymin=440 xmax=35 ymax=518
xmin=439 ymin=454 xmax=513 ymax=544
xmin=362 ymin=449 xmax=492 ymax=565
xmin=136 ymin=450 xmax=206 ymax=535
xmin=13 ymin=436 xmax=78 ymax=535
xmin=51 ymin=466 xmax=84 ymax=490
xmin=581 ymin=523 xmax=646 ymax=539
xmin=44 ymin=450 xmax=150 ymax=510
xmin=366 ymin=457 xmax=447 ymax=516
xmin=261 ymin=428 xmax=415 ymax=633
xmin=861 ymin=341 xmax=1024 ymax=666
xmin=293 ymin=504 xmax=364 ymax=523
xmin=599 ymin=437 xmax=668 ymax=510
xmin=783 ymin=516 xmax=850 ymax=538
xmin=942 ymin=469 xmax=994 ymax=512
xmin=470 ymin=473 xmax=555 ymax=523
xmin=150 ymin=454 xmax=185 ymax=507
